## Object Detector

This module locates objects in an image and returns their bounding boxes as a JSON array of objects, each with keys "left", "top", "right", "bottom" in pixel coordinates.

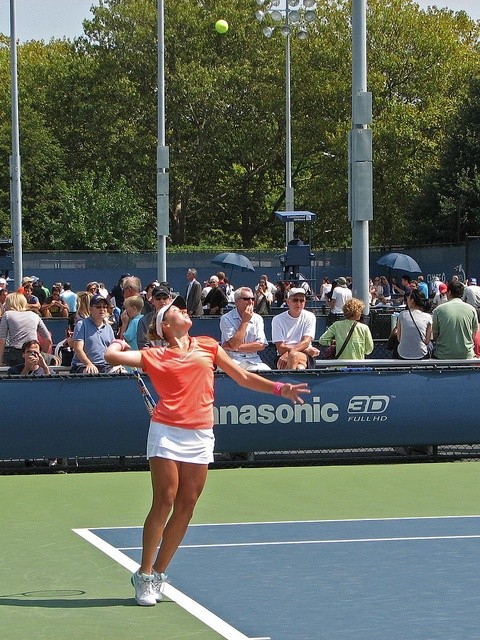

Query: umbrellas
[
  {"left": 211, "top": 252, "right": 256, "bottom": 285},
  {"left": 376, "top": 252, "right": 422, "bottom": 273}
]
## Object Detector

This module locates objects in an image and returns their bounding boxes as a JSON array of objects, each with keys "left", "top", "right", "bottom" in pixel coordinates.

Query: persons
[
  {"left": 320, "top": 277, "right": 352, "bottom": 331},
  {"left": 272, "top": 286, "right": 318, "bottom": 372},
  {"left": 255, "top": 273, "right": 277, "bottom": 314},
  {"left": 214, "top": 272, "right": 230, "bottom": 299},
  {"left": 219, "top": 286, "right": 272, "bottom": 370},
  {"left": 0, "top": 275, "right": 121, "bottom": 310},
  {"left": 69, "top": 294, "right": 125, "bottom": 377},
  {"left": 463, "top": 279, "right": 480, "bottom": 315},
  {"left": 137, "top": 285, "right": 171, "bottom": 348},
  {"left": 394, "top": 289, "right": 432, "bottom": 362},
  {"left": 119, "top": 277, "right": 144, "bottom": 329},
  {"left": 370, "top": 277, "right": 448, "bottom": 303},
  {"left": 319, "top": 297, "right": 375, "bottom": 362},
  {"left": 104, "top": 296, "right": 310, "bottom": 606},
  {"left": 432, "top": 278, "right": 479, "bottom": 365},
  {"left": 1, "top": 295, "right": 52, "bottom": 363},
  {"left": 278, "top": 228, "right": 303, "bottom": 280},
  {"left": 59, "top": 313, "right": 79, "bottom": 366},
  {"left": 142, "top": 279, "right": 161, "bottom": 314},
  {"left": 106, "top": 303, "right": 121, "bottom": 341},
  {"left": 185, "top": 268, "right": 204, "bottom": 317},
  {"left": 118, "top": 296, "right": 145, "bottom": 373},
  {"left": 10, "top": 340, "right": 52, "bottom": 378},
  {"left": 112, "top": 272, "right": 129, "bottom": 308},
  {"left": 275, "top": 280, "right": 313, "bottom": 306},
  {"left": 202, "top": 276, "right": 227, "bottom": 316}
]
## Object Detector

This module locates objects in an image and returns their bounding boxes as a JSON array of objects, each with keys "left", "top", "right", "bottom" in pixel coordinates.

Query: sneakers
[
  {"left": 131, "top": 567, "right": 157, "bottom": 606},
  {"left": 151, "top": 567, "right": 168, "bottom": 601}
]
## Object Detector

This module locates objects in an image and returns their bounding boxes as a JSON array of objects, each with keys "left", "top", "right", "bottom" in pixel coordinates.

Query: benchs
[
  {"left": 0, "top": 365, "right": 72, "bottom": 372},
  {"left": 314, "top": 358, "right": 480, "bottom": 364}
]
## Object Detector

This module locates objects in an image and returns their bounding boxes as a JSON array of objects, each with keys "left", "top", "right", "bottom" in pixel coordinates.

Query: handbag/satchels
[
  {"left": 325, "top": 321, "right": 358, "bottom": 359},
  {"left": 409, "top": 309, "right": 434, "bottom": 359}
]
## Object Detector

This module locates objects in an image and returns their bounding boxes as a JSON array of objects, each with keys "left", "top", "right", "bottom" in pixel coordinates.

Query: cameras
[{"left": 28, "top": 349, "right": 37, "bottom": 362}]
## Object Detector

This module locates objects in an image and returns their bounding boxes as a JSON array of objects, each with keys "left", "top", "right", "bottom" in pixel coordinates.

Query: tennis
[{"left": 215, "top": 20, "right": 228, "bottom": 34}]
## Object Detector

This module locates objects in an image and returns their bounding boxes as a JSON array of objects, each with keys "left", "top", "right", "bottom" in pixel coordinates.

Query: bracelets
[
  {"left": 112, "top": 339, "right": 125, "bottom": 350},
  {"left": 272, "top": 381, "right": 285, "bottom": 396}
]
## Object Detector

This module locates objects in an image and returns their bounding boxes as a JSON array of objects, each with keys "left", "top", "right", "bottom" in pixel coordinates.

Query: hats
[
  {"left": 287, "top": 288, "right": 306, "bottom": 298},
  {"left": 156, "top": 295, "right": 186, "bottom": 339},
  {"left": 334, "top": 277, "right": 347, "bottom": 288},
  {"left": 90, "top": 295, "right": 107, "bottom": 305},
  {"left": 30, "top": 275, "right": 40, "bottom": 284},
  {"left": 22, "top": 277, "right": 32, "bottom": 286},
  {"left": 439, "top": 284, "right": 447, "bottom": 294},
  {"left": 470, "top": 278, "right": 476, "bottom": 284},
  {"left": 152, "top": 286, "right": 170, "bottom": 298}
]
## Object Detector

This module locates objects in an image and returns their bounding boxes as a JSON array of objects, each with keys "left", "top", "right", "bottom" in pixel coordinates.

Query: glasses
[
  {"left": 155, "top": 298, "right": 167, "bottom": 300},
  {"left": 238, "top": 297, "right": 255, "bottom": 301},
  {"left": 91, "top": 305, "right": 108, "bottom": 308},
  {"left": 293, "top": 299, "right": 305, "bottom": 303}
]
